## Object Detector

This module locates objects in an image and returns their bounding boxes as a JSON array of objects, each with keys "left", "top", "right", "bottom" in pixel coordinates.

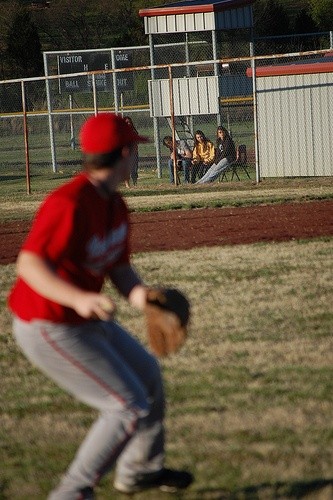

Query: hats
[{"left": 79, "top": 112, "right": 150, "bottom": 156}]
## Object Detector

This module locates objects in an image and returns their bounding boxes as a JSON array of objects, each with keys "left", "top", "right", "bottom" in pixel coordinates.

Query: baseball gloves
[{"left": 146, "top": 286, "right": 190, "bottom": 354}]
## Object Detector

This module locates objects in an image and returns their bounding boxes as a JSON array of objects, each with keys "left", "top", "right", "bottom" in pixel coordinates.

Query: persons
[
  {"left": 6, "top": 114, "right": 195, "bottom": 500},
  {"left": 119, "top": 116, "right": 139, "bottom": 190},
  {"left": 192, "top": 125, "right": 236, "bottom": 185},
  {"left": 188, "top": 130, "right": 215, "bottom": 183},
  {"left": 161, "top": 135, "right": 191, "bottom": 186}
]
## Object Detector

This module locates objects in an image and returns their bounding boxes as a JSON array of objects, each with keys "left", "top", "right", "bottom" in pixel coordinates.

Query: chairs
[{"left": 175, "top": 144, "right": 251, "bottom": 182}]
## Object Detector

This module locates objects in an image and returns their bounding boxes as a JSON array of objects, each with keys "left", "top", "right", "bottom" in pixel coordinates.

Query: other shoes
[{"left": 115, "top": 460, "right": 194, "bottom": 494}]
